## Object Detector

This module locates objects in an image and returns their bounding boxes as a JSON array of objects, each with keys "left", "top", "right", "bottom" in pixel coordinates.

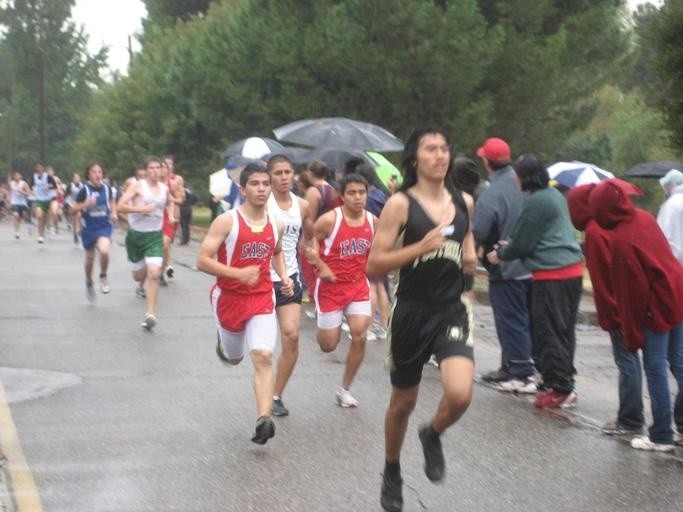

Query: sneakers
[
  {"left": 142, "top": 313, "right": 155, "bottom": 329},
  {"left": 482, "top": 370, "right": 577, "bottom": 408},
  {"left": 99, "top": 274, "right": 110, "bottom": 294},
  {"left": 381, "top": 473, "right": 402, "bottom": 511},
  {"left": 272, "top": 399, "right": 288, "bottom": 416},
  {"left": 603, "top": 421, "right": 674, "bottom": 452},
  {"left": 336, "top": 392, "right": 358, "bottom": 408},
  {"left": 419, "top": 425, "right": 443, "bottom": 481},
  {"left": 252, "top": 416, "right": 273, "bottom": 445}
]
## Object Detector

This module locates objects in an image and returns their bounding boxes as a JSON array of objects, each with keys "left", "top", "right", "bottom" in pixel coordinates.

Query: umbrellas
[{"left": 272, "top": 116, "right": 405, "bottom": 153}]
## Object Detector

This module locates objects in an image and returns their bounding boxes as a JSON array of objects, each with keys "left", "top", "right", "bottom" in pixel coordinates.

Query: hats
[{"left": 476, "top": 138, "right": 510, "bottom": 161}]
